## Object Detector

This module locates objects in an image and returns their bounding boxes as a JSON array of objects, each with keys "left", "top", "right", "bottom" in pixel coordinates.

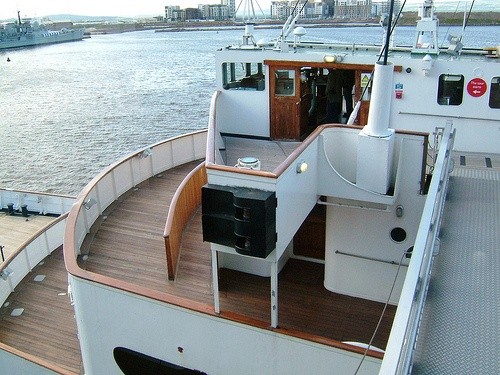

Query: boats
[{"left": 0, "top": 10, "right": 86, "bottom": 49}]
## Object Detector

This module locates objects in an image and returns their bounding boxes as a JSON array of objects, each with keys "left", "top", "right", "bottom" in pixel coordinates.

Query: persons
[{"left": 322, "top": 69, "right": 355, "bottom": 124}]
